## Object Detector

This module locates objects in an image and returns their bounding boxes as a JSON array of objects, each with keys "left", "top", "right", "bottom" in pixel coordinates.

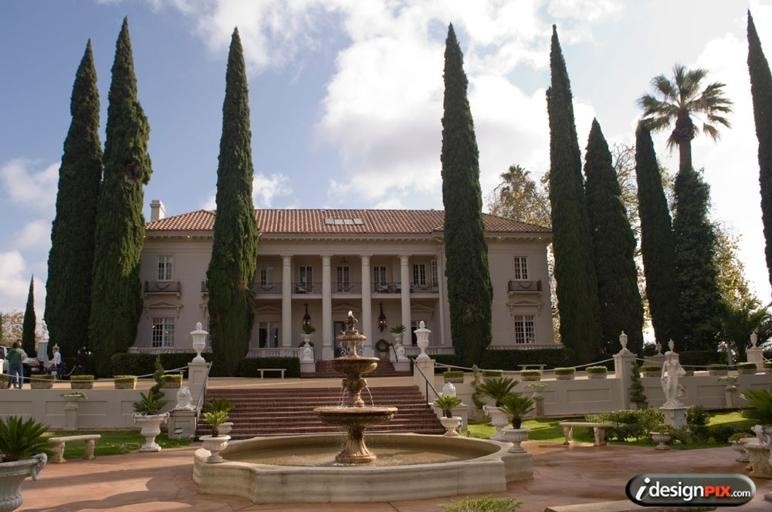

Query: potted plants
[
  {"left": 687, "top": 407, "right": 710, "bottom": 444},
  {"left": 649, "top": 425, "right": 674, "bottom": 450},
  {"left": 728, "top": 387, "right": 772, "bottom": 502},
  {"left": 390, "top": 324, "right": 407, "bottom": 345},
  {"left": 199, "top": 398, "right": 235, "bottom": 464},
  {"left": 433, "top": 394, "right": 462, "bottom": 436},
  {"left": 132, "top": 392, "right": 169, "bottom": 453},
  {"left": 301, "top": 325, "right": 315, "bottom": 346},
  {"left": 479, "top": 377, "right": 535, "bottom": 454},
  {"left": 0, "top": 414, "right": 49, "bottom": 512}
]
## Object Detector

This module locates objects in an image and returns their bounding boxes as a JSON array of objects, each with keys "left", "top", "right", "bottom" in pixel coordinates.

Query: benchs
[
  {"left": 561, "top": 422, "right": 611, "bottom": 448},
  {"left": 50, "top": 434, "right": 101, "bottom": 463},
  {"left": 257, "top": 368, "right": 287, "bottom": 380},
  {"left": 517, "top": 364, "right": 548, "bottom": 371}
]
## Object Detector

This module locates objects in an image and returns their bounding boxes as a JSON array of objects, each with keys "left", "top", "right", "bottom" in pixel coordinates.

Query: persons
[
  {"left": 660, "top": 350, "right": 686, "bottom": 407},
  {"left": 13, "top": 339, "right": 23, "bottom": 388},
  {"left": 42, "top": 344, "right": 63, "bottom": 370},
  {"left": 5, "top": 342, "right": 26, "bottom": 389}
]
